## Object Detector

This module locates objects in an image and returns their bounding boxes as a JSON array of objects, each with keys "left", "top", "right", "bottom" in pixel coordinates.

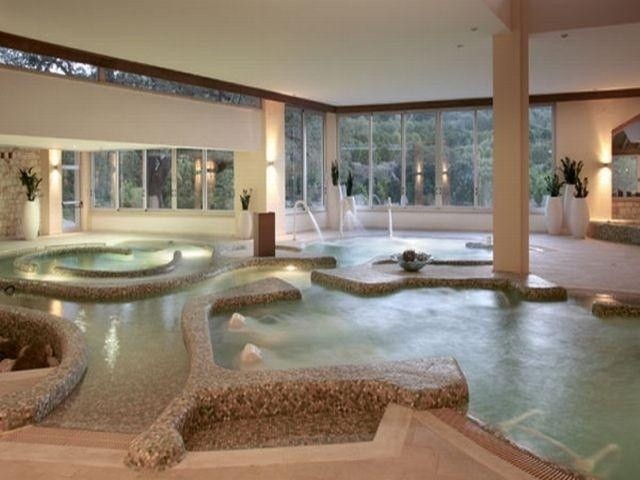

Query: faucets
[
  {"left": 386, "top": 196, "right": 394, "bottom": 231},
  {"left": 292, "top": 199, "right": 309, "bottom": 240}
]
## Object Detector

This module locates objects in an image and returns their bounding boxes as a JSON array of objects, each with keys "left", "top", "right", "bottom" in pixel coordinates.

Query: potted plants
[
  {"left": 326, "top": 159, "right": 356, "bottom": 230},
  {"left": 544, "top": 157, "right": 589, "bottom": 238},
  {"left": 239, "top": 186, "right": 252, "bottom": 240},
  {"left": 17, "top": 164, "right": 43, "bottom": 240}
]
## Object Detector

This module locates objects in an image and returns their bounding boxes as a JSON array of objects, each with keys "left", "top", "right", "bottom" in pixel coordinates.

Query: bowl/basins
[{"left": 391, "top": 254, "right": 433, "bottom": 272}]
